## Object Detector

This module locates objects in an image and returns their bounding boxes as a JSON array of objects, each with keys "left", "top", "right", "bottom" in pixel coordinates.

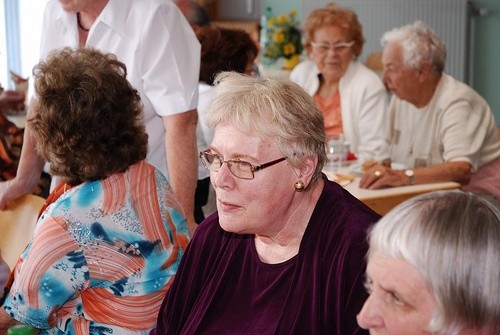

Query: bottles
[{"left": 7, "top": 324, "right": 36, "bottom": 335}]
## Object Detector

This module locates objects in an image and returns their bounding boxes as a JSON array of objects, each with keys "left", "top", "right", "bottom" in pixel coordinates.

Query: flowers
[{"left": 262, "top": 10, "right": 304, "bottom": 59}]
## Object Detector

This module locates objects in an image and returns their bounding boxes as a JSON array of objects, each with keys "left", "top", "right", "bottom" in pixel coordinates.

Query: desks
[{"left": 323, "top": 156, "right": 460, "bottom": 217}]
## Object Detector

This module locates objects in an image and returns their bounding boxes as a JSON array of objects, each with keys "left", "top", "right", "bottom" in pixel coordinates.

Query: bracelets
[{"left": 77, "top": 12, "right": 91, "bottom": 32}]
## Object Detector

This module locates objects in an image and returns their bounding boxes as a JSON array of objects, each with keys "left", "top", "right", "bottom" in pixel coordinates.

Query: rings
[{"left": 375, "top": 171, "right": 381, "bottom": 177}]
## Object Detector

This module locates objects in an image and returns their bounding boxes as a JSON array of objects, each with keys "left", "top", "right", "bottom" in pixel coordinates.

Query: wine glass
[{"left": 326, "top": 131, "right": 343, "bottom": 175}]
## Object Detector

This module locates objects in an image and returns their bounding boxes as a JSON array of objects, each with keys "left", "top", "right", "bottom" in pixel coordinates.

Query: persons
[
  {"left": 0, "top": 0, "right": 221, "bottom": 242},
  {"left": 0, "top": 4, "right": 500, "bottom": 335}
]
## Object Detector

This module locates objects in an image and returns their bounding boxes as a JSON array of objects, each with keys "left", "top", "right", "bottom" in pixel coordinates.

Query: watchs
[{"left": 403, "top": 167, "right": 413, "bottom": 185}]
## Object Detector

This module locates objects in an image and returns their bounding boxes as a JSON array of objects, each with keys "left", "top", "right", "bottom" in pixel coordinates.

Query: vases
[{"left": 283, "top": 55, "right": 300, "bottom": 70}]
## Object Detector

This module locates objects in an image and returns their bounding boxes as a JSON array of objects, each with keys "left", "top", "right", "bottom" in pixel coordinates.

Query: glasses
[
  {"left": 199, "top": 149, "right": 304, "bottom": 180},
  {"left": 246, "top": 62, "right": 259, "bottom": 77},
  {"left": 310, "top": 41, "right": 355, "bottom": 57}
]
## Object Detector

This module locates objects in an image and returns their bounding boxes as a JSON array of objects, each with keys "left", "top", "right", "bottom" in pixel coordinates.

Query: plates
[{"left": 348, "top": 162, "right": 409, "bottom": 176}]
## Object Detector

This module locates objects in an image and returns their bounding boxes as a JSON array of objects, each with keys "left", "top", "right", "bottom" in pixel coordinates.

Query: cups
[
  {"left": 413, "top": 149, "right": 433, "bottom": 171},
  {"left": 343, "top": 128, "right": 355, "bottom": 161}
]
